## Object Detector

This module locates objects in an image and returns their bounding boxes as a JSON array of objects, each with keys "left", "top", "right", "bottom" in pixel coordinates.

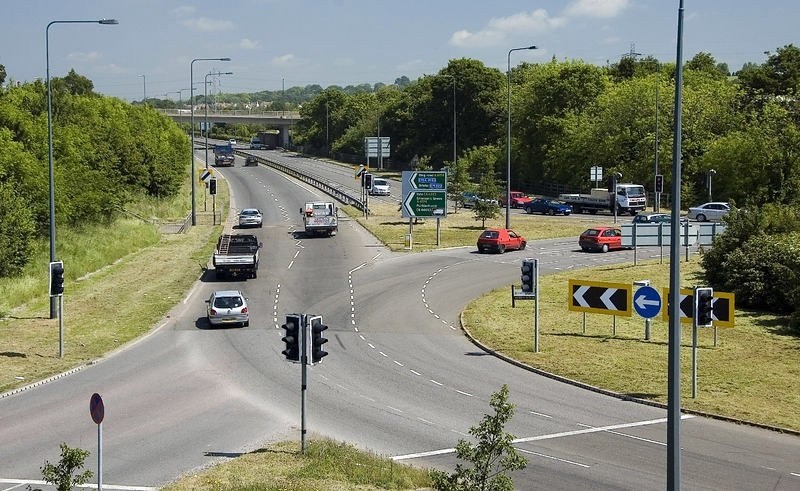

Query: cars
[
  {"left": 454, "top": 182, "right": 749, "bottom": 224},
  {"left": 213, "top": 137, "right": 262, "bottom": 168},
  {"left": 578, "top": 226, "right": 635, "bottom": 254},
  {"left": 477, "top": 228, "right": 527, "bottom": 255},
  {"left": 203, "top": 290, "right": 250, "bottom": 327},
  {"left": 237, "top": 208, "right": 263, "bottom": 228},
  {"left": 368, "top": 177, "right": 391, "bottom": 196}
]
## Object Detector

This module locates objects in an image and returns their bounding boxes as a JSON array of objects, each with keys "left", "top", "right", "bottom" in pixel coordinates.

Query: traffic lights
[
  {"left": 210, "top": 178, "right": 217, "bottom": 195},
  {"left": 281, "top": 315, "right": 302, "bottom": 363},
  {"left": 49, "top": 261, "right": 65, "bottom": 297},
  {"left": 521, "top": 260, "right": 535, "bottom": 293},
  {"left": 654, "top": 175, "right": 664, "bottom": 193},
  {"left": 364, "top": 174, "right": 372, "bottom": 190},
  {"left": 309, "top": 315, "right": 329, "bottom": 365},
  {"left": 695, "top": 287, "right": 713, "bottom": 328}
]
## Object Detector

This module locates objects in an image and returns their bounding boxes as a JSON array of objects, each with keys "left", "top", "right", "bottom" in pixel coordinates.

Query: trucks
[
  {"left": 299, "top": 200, "right": 339, "bottom": 239},
  {"left": 213, "top": 233, "right": 264, "bottom": 280}
]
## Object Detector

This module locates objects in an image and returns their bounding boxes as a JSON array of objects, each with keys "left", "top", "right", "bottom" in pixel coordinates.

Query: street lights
[
  {"left": 204, "top": 72, "right": 235, "bottom": 171},
  {"left": 506, "top": 45, "right": 540, "bottom": 228},
  {"left": 166, "top": 81, "right": 213, "bottom": 144},
  {"left": 137, "top": 74, "right": 146, "bottom": 101},
  {"left": 45, "top": 17, "right": 119, "bottom": 321},
  {"left": 190, "top": 57, "right": 231, "bottom": 228},
  {"left": 436, "top": 74, "right": 458, "bottom": 182}
]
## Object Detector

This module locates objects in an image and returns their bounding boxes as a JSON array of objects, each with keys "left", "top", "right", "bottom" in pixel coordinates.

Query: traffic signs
[{"left": 401, "top": 171, "right": 447, "bottom": 219}]
